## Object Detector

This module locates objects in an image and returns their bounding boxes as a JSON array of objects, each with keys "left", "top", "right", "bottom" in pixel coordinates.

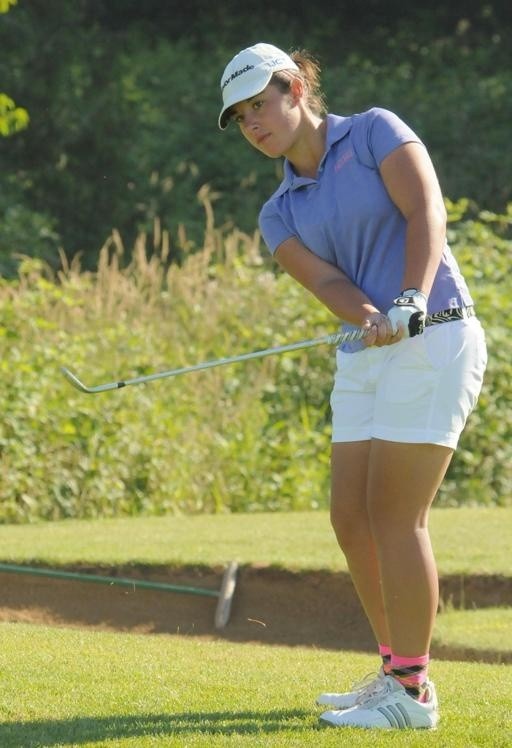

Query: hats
[{"left": 217, "top": 42, "right": 299, "bottom": 131}]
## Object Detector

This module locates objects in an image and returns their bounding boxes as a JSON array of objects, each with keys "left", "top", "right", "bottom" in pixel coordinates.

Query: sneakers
[
  {"left": 318, "top": 675, "right": 437, "bottom": 729},
  {"left": 317, "top": 664, "right": 386, "bottom": 707}
]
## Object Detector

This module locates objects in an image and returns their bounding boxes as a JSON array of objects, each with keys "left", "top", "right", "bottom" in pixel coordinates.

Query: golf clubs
[{"left": 61, "top": 304, "right": 476, "bottom": 393}]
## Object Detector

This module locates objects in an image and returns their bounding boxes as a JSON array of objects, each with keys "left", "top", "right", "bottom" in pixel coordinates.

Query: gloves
[{"left": 387, "top": 288, "right": 428, "bottom": 338}]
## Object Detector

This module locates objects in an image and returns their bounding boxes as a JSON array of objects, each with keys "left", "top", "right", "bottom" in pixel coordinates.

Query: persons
[{"left": 215, "top": 42, "right": 491, "bottom": 731}]
